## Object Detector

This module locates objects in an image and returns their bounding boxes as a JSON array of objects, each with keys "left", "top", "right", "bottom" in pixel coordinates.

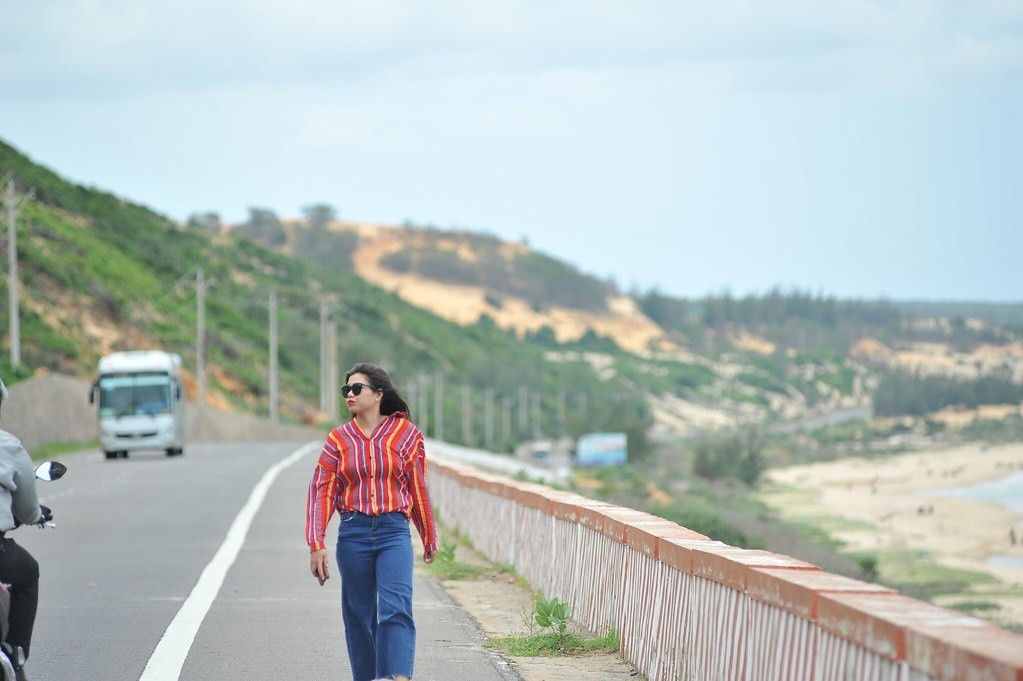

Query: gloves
[{"left": 37, "top": 504, "right": 54, "bottom": 524}]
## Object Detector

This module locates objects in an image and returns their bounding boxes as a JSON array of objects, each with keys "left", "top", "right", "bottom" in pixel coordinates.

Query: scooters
[{"left": 0, "top": 461, "right": 68, "bottom": 643}]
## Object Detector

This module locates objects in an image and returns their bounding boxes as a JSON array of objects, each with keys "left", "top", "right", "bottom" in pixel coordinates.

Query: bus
[{"left": 89, "top": 350, "right": 183, "bottom": 458}]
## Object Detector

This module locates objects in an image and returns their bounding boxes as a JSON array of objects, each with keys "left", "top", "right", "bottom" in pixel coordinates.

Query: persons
[
  {"left": 304, "top": 364, "right": 439, "bottom": 681},
  {"left": 0, "top": 377, "right": 53, "bottom": 669}
]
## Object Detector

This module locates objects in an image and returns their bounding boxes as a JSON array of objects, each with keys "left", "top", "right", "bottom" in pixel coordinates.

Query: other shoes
[{"left": 0, "top": 642, "right": 25, "bottom": 681}]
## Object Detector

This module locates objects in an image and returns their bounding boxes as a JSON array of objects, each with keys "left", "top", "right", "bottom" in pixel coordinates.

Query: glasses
[{"left": 342, "top": 383, "right": 378, "bottom": 398}]
